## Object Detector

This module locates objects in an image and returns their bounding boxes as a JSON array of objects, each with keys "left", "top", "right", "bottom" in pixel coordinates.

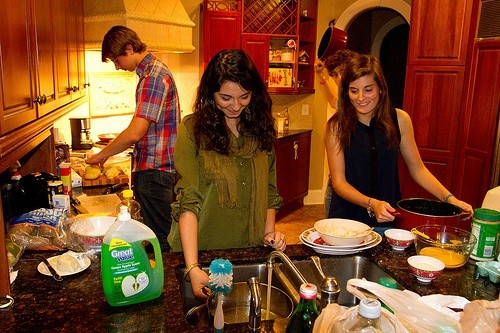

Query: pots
[
  {"left": 317, "top": 16, "right": 348, "bottom": 69},
  {"left": 388, "top": 196, "right": 471, "bottom": 239},
  {"left": 202, "top": 282, "right": 294, "bottom": 325}
]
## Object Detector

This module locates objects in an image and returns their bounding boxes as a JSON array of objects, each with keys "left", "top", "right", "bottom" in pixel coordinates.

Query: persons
[
  {"left": 324, "top": 49, "right": 358, "bottom": 218},
  {"left": 85, "top": 26, "right": 181, "bottom": 252},
  {"left": 326, "top": 55, "right": 473, "bottom": 228},
  {"left": 166, "top": 49, "right": 286, "bottom": 298}
]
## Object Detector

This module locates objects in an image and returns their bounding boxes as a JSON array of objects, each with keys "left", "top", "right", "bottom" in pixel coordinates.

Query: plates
[
  {"left": 37, "top": 255, "right": 91, "bottom": 279},
  {"left": 302, "top": 227, "right": 377, "bottom": 249},
  {"left": 299, "top": 233, "right": 381, "bottom": 255}
]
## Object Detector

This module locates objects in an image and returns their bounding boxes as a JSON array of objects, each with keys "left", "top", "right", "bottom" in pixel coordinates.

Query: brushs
[{"left": 207, "top": 258, "right": 233, "bottom": 333}]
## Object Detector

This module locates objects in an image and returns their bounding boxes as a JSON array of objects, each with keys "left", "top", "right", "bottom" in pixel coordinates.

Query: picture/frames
[{"left": 87, "top": 71, "right": 137, "bottom": 119}]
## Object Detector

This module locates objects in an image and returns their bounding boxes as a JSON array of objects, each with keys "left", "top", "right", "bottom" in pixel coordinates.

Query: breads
[{"left": 6, "top": 210, "right": 78, "bottom": 249}]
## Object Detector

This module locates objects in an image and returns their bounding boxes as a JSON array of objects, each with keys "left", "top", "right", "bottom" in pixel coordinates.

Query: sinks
[
  {"left": 279, "top": 255, "right": 416, "bottom": 311},
  {"left": 173, "top": 262, "right": 301, "bottom": 324}
]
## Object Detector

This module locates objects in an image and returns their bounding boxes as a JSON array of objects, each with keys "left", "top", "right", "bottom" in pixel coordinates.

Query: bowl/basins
[
  {"left": 97, "top": 133, "right": 122, "bottom": 141},
  {"left": 71, "top": 216, "right": 116, "bottom": 245},
  {"left": 313, "top": 217, "right": 477, "bottom": 283}
]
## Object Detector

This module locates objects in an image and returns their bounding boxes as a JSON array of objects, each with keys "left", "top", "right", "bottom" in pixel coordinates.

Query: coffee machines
[{"left": 69, "top": 117, "right": 95, "bottom": 149}]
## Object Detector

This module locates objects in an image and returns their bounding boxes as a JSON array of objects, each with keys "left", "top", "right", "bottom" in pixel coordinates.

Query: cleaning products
[
  {"left": 100, "top": 204, "right": 165, "bottom": 306},
  {"left": 116, "top": 189, "right": 144, "bottom": 223}
]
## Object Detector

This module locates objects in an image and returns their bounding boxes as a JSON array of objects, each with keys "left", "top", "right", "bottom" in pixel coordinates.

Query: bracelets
[
  {"left": 183, "top": 263, "right": 202, "bottom": 283},
  {"left": 366, "top": 197, "right": 375, "bottom": 218},
  {"left": 445, "top": 194, "right": 453, "bottom": 203},
  {"left": 262, "top": 232, "right": 273, "bottom": 240}
]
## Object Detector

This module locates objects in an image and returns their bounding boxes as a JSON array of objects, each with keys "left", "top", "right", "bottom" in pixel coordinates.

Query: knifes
[
  {"left": 70, "top": 194, "right": 89, "bottom": 214},
  {"left": 34, "top": 253, "right": 63, "bottom": 281}
]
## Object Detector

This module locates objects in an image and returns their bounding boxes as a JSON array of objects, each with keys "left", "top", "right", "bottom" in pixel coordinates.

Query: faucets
[
  {"left": 267, "top": 251, "right": 341, "bottom": 315},
  {"left": 245, "top": 276, "right": 262, "bottom": 329}
]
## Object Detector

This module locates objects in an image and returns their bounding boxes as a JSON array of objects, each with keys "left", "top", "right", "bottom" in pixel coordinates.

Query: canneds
[{"left": 465, "top": 208, "right": 500, "bottom": 262}]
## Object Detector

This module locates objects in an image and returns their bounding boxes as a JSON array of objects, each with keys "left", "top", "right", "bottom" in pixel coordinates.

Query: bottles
[
  {"left": 101, "top": 206, "right": 164, "bottom": 307},
  {"left": 346, "top": 297, "right": 382, "bottom": 333},
  {"left": 284, "top": 107, "right": 289, "bottom": 130},
  {"left": 285, "top": 283, "right": 320, "bottom": 333},
  {"left": 468, "top": 208, "right": 500, "bottom": 259},
  {"left": 115, "top": 189, "right": 145, "bottom": 247},
  {"left": 375, "top": 277, "right": 397, "bottom": 315}
]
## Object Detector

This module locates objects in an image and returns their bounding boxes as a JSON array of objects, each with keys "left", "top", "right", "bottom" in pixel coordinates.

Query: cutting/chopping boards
[
  {"left": 76, "top": 194, "right": 121, "bottom": 217},
  {"left": 78, "top": 166, "right": 130, "bottom": 189}
]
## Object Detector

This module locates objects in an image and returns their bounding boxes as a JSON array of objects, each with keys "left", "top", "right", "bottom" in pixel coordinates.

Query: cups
[{"left": 281, "top": 47, "right": 294, "bottom": 62}]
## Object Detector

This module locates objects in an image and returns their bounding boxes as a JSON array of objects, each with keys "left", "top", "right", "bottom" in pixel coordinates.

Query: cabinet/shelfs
[
  {"left": 275, "top": 133, "right": 309, "bottom": 223},
  {"left": 0, "top": 0, "right": 87, "bottom": 174},
  {"left": 397, "top": 0, "right": 500, "bottom": 208},
  {"left": 199, "top": 0, "right": 318, "bottom": 96}
]
